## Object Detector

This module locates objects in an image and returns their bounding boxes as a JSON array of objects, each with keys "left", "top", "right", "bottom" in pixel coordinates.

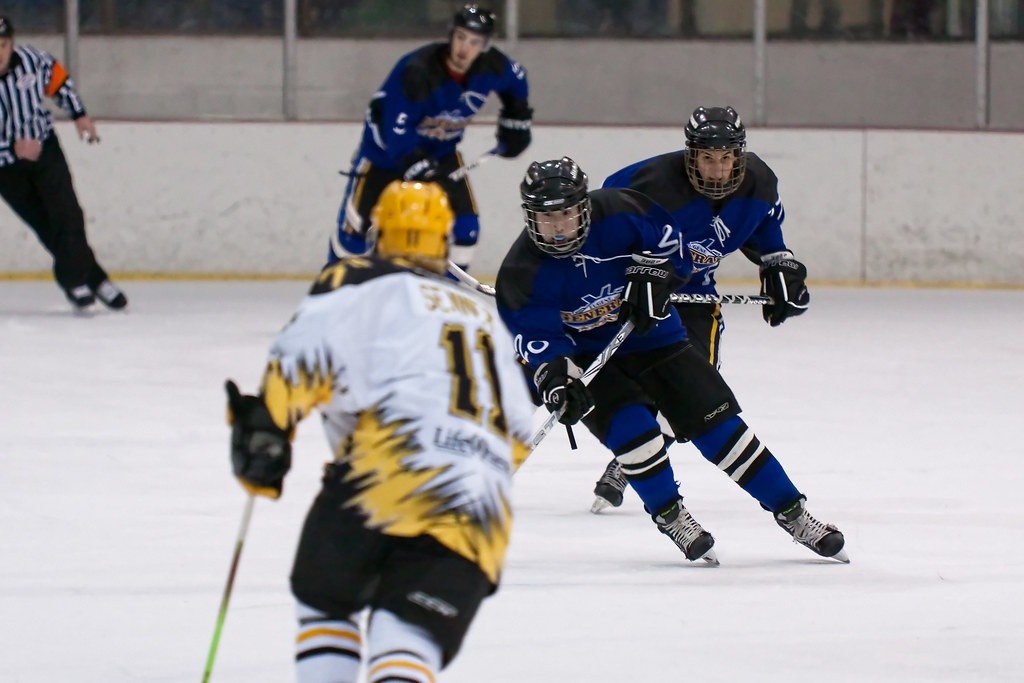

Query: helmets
[
  {"left": 520, "top": 157, "right": 592, "bottom": 260},
  {"left": 684, "top": 106, "right": 746, "bottom": 200},
  {"left": 448, "top": 5, "right": 496, "bottom": 52},
  {"left": 369, "top": 179, "right": 453, "bottom": 259},
  {"left": 0, "top": 15, "right": 13, "bottom": 36}
]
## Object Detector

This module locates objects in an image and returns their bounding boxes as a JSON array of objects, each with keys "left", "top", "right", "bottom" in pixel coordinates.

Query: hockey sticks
[
  {"left": 201, "top": 487, "right": 261, "bottom": 683},
  {"left": 441, "top": 149, "right": 504, "bottom": 194},
  {"left": 668, "top": 290, "right": 780, "bottom": 305},
  {"left": 514, "top": 317, "right": 636, "bottom": 461},
  {"left": 448, "top": 256, "right": 494, "bottom": 295}
]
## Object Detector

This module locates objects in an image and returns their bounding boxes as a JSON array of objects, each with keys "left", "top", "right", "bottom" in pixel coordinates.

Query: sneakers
[
  {"left": 592, "top": 457, "right": 628, "bottom": 513},
  {"left": 760, "top": 489, "right": 851, "bottom": 563},
  {"left": 644, "top": 493, "right": 719, "bottom": 565}
]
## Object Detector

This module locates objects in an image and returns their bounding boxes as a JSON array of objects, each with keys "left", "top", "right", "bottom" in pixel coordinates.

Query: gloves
[
  {"left": 401, "top": 152, "right": 444, "bottom": 184},
  {"left": 498, "top": 109, "right": 532, "bottom": 156},
  {"left": 758, "top": 259, "right": 809, "bottom": 326},
  {"left": 532, "top": 357, "right": 596, "bottom": 424},
  {"left": 619, "top": 254, "right": 677, "bottom": 327}
]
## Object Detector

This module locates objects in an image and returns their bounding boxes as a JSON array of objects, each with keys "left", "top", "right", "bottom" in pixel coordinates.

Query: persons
[
  {"left": 0, "top": 15, "right": 127, "bottom": 314},
  {"left": 222, "top": 180, "right": 533, "bottom": 683},
  {"left": 327, "top": 3, "right": 534, "bottom": 283},
  {"left": 494, "top": 156, "right": 850, "bottom": 566},
  {"left": 590, "top": 105, "right": 810, "bottom": 515}
]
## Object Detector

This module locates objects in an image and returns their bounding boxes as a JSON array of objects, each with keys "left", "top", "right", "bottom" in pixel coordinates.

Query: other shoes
[
  {"left": 94, "top": 279, "right": 127, "bottom": 307},
  {"left": 66, "top": 282, "right": 94, "bottom": 308}
]
